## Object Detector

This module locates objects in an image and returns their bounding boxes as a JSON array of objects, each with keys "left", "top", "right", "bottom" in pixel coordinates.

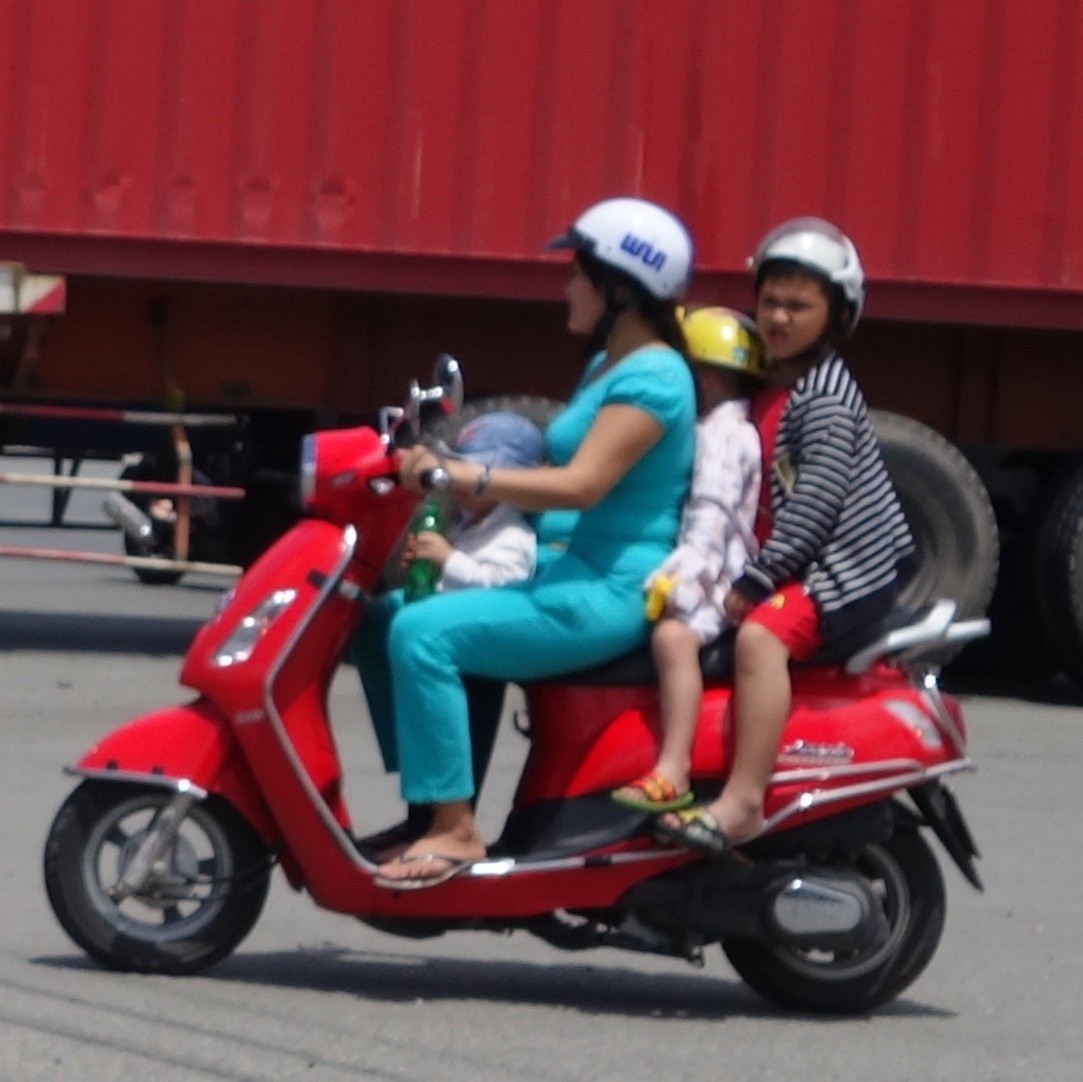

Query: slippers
[
  {"left": 361, "top": 821, "right": 413, "bottom": 850},
  {"left": 613, "top": 777, "right": 698, "bottom": 812},
  {"left": 655, "top": 804, "right": 755, "bottom": 870},
  {"left": 372, "top": 842, "right": 474, "bottom": 889}
]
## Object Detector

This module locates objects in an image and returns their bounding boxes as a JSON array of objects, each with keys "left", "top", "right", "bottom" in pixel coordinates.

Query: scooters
[
  {"left": 107, "top": 409, "right": 379, "bottom": 586},
  {"left": 43, "top": 348, "right": 994, "bottom": 1021}
]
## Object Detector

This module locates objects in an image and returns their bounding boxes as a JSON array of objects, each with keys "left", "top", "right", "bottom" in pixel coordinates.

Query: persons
[
  {"left": 610, "top": 303, "right": 766, "bottom": 812},
  {"left": 346, "top": 197, "right": 695, "bottom": 887},
  {"left": 374, "top": 414, "right": 545, "bottom": 846},
  {"left": 655, "top": 219, "right": 914, "bottom": 875}
]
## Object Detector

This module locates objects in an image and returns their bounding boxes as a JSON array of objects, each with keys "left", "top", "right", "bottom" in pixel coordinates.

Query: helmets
[
  {"left": 752, "top": 217, "right": 863, "bottom": 334},
  {"left": 436, "top": 410, "right": 542, "bottom": 478},
  {"left": 550, "top": 196, "right": 693, "bottom": 304},
  {"left": 683, "top": 307, "right": 766, "bottom": 381}
]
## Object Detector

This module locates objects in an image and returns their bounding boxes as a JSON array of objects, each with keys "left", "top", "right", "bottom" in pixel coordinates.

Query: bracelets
[{"left": 473, "top": 466, "right": 490, "bottom": 496}]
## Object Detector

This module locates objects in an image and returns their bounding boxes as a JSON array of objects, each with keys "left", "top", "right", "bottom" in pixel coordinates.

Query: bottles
[
  {"left": 646, "top": 578, "right": 665, "bottom": 620},
  {"left": 405, "top": 499, "right": 443, "bottom": 605}
]
